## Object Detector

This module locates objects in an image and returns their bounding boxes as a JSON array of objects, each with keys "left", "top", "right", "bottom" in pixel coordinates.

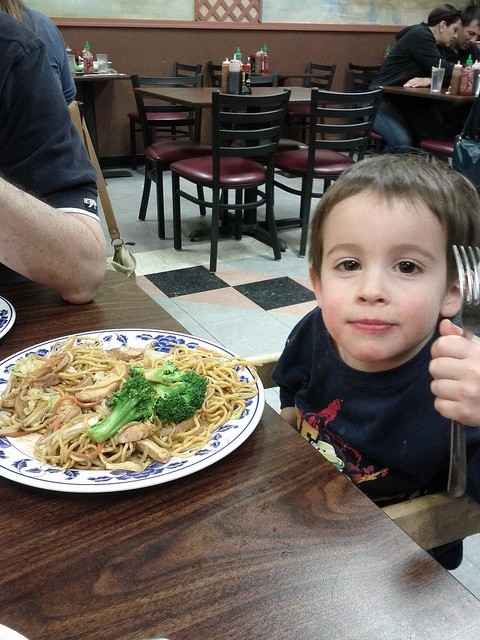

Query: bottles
[
  {"left": 462, "top": 67, "right": 474, "bottom": 95},
  {"left": 234, "top": 46, "right": 242, "bottom": 60},
  {"left": 75, "top": 50, "right": 84, "bottom": 76},
  {"left": 245, "top": 44, "right": 269, "bottom": 77},
  {"left": 450, "top": 64, "right": 461, "bottom": 96},
  {"left": 66, "top": 43, "right": 76, "bottom": 77},
  {"left": 228, "top": 55, "right": 241, "bottom": 95},
  {"left": 82, "top": 40, "right": 95, "bottom": 75},
  {"left": 241, "top": 64, "right": 252, "bottom": 95},
  {"left": 221, "top": 57, "right": 229, "bottom": 93}
]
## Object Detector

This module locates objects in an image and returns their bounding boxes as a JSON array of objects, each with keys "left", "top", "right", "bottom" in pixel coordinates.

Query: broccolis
[
  {"left": 129, "top": 360, "right": 208, "bottom": 425},
  {"left": 88, "top": 376, "right": 156, "bottom": 442}
]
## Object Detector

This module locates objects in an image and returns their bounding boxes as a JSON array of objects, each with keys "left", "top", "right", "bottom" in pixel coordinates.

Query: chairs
[
  {"left": 126, "top": 60, "right": 203, "bottom": 171},
  {"left": 207, "top": 59, "right": 247, "bottom": 148},
  {"left": 274, "top": 83, "right": 386, "bottom": 259},
  {"left": 346, "top": 61, "right": 386, "bottom": 154},
  {"left": 169, "top": 87, "right": 293, "bottom": 275},
  {"left": 417, "top": 88, "right": 480, "bottom": 169},
  {"left": 127, "top": 71, "right": 207, "bottom": 241},
  {"left": 287, "top": 60, "right": 338, "bottom": 149}
]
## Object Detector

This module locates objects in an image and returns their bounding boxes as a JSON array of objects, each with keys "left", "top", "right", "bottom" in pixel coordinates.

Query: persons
[
  {"left": 442, "top": 5, "right": 480, "bottom": 65},
  {"left": 272, "top": 157, "right": 480, "bottom": 570},
  {"left": 362, "top": 4, "right": 462, "bottom": 155},
  {"left": 0, "top": 0, "right": 77, "bottom": 105},
  {"left": 0, "top": 14, "right": 106, "bottom": 305}
]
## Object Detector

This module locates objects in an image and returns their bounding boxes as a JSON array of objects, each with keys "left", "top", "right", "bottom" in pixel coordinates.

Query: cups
[
  {"left": 97, "top": 54, "right": 110, "bottom": 74},
  {"left": 431, "top": 67, "right": 445, "bottom": 95}
]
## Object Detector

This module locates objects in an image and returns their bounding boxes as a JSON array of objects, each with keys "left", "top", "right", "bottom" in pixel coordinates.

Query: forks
[{"left": 446, "top": 244, "right": 480, "bottom": 499}]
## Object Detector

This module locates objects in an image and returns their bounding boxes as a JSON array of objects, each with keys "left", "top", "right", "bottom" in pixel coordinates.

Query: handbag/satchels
[
  {"left": 67, "top": 101, "right": 138, "bottom": 284},
  {"left": 451, "top": 92, "right": 480, "bottom": 191}
]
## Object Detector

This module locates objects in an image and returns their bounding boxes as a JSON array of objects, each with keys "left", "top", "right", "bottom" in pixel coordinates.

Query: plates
[{"left": 0, "top": 293, "right": 16, "bottom": 339}]
